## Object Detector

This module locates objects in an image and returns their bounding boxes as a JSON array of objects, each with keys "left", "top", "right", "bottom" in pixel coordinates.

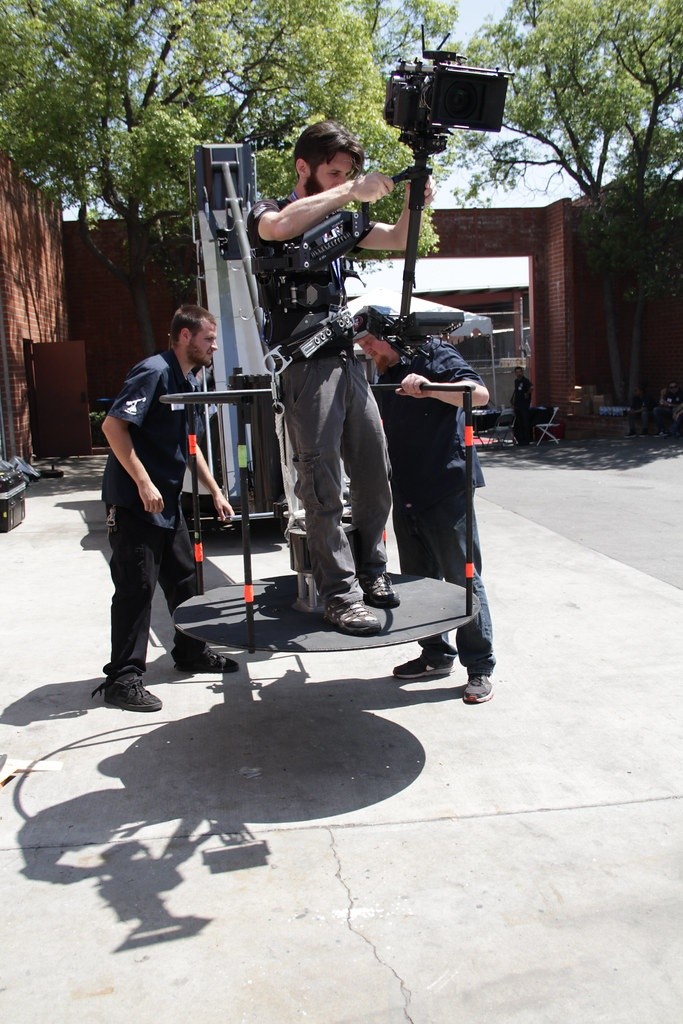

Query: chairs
[
  {"left": 473, "top": 411, "right": 518, "bottom": 449},
  {"left": 534, "top": 406, "right": 561, "bottom": 447}
]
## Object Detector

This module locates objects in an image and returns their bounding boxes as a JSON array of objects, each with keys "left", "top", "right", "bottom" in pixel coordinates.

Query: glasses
[{"left": 669, "top": 385, "right": 677, "bottom": 389}]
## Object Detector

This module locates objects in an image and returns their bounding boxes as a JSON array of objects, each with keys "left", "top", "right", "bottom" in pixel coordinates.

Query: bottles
[{"left": 599, "top": 406, "right": 630, "bottom": 416}]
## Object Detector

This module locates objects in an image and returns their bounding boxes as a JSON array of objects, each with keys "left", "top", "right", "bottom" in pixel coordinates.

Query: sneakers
[
  {"left": 360, "top": 573, "right": 401, "bottom": 608},
  {"left": 393, "top": 658, "right": 454, "bottom": 679},
  {"left": 174, "top": 652, "right": 238, "bottom": 673},
  {"left": 324, "top": 601, "right": 382, "bottom": 636},
  {"left": 91, "top": 672, "right": 163, "bottom": 711},
  {"left": 464, "top": 674, "right": 494, "bottom": 702}
]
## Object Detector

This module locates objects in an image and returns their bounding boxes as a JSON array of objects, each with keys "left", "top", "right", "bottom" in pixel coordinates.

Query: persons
[
  {"left": 351, "top": 305, "right": 496, "bottom": 703},
  {"left": 247, "top": 120, "right": 437, "bottom": 635},
  {"left": 624, "top": 381, "right": 683, "bottom": 438},
  {"left": 510, "top": 366, "right": 534, "bottom": 446},
  {"left": 102, "top": 305, "right": 236, "bottom": 712}
]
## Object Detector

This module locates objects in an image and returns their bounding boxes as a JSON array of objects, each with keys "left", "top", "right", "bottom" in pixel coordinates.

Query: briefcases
[{"left": 0, "top": 468, "right": 26, "bottom": 533}]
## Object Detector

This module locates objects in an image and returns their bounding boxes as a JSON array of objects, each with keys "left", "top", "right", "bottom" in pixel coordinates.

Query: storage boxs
[
  {"left": 570, "top": 386, "right": 614, "bottom": 415},
  {"left": 0, "top": 469, "right": 26, "bottom": 532}
]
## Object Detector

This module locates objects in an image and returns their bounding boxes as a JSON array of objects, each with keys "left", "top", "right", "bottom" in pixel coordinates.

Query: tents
[{"left": 347, "top": 290, "right": 500, "bottom": 443}]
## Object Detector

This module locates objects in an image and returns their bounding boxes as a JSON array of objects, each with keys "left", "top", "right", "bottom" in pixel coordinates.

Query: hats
[{"left": 349, "top": 304, "right": 391, "bottom": 341}]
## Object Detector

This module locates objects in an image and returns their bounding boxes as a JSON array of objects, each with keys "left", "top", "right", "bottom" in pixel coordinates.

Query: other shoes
[
  {"left": 624, "top": 430, "right": 637, "bottom": 438},
  {"left": 652, "top": 429, "right": 665, "bottom": 437},
  {"left": 639, "top": 429, "right": 649, "bottom": 437}
]
